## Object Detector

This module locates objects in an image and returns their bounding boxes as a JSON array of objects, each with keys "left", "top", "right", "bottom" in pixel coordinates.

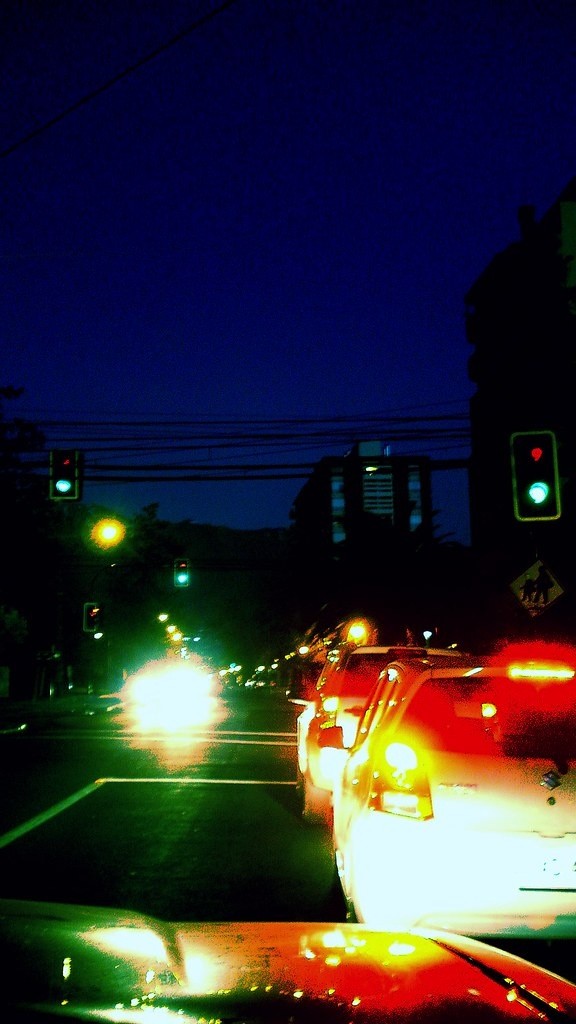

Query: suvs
[
  {"left": 295, "top": 646, "right": 467, "bottom": 822},
  {"left": 334, "top": 656, "right": 576, "bottom": 942}
]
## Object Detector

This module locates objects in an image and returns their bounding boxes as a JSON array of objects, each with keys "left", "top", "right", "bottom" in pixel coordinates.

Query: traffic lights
[
  {"left": 83, "top": 602, "right": 99, "bottom": 632},
  {"left": 509, "top": 430, "right": 561, "bottom": 520},
  {"left": 48, "top": 448, "right": 80, "bottom": 500},
  {"left": 174, "top": 557, "right": 190, "bottom": 587}
]
